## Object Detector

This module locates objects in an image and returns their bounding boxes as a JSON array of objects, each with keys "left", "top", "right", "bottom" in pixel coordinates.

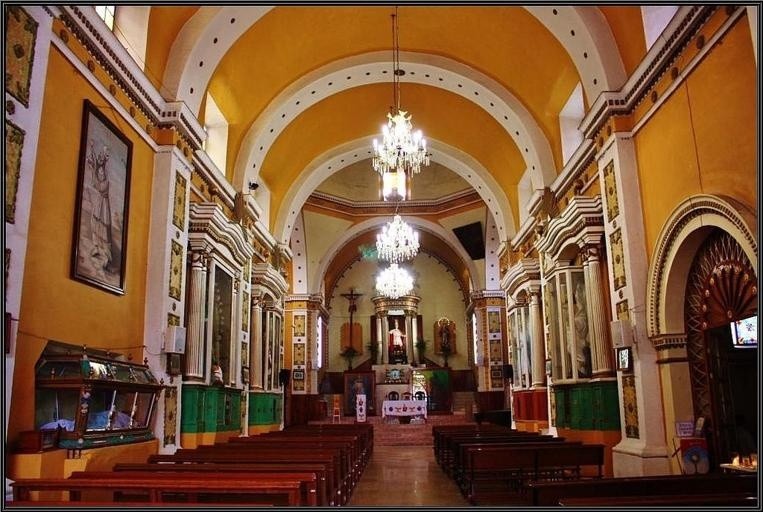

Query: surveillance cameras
[{"left": 249, "top": 176, "right": 259, "bottom": 190}]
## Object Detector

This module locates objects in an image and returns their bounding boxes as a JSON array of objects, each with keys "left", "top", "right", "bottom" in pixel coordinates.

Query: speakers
[
  {"left": 279, "top": 368, "right": 291, "bottom": 381},
  {"left": 609, "top": 319, "right": 633, "bottom": 349},
  {"left": 162, "top": 324, "right": 188, "bottom": 355},
  {"left": 502, "top": 364, "right": 514, "bottom": 380}
]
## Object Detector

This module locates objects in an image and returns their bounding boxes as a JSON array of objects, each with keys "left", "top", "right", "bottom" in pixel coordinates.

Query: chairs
[{"left": 388, "top": 390, "right": 425, "bottom": 401}]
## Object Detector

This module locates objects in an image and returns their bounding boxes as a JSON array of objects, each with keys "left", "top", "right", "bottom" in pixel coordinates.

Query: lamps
[{"left": 371, "top": 4, "right": 432, "bottom": 301}]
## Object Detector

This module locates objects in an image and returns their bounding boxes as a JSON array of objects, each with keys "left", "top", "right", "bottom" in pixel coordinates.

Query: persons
[
  {"left": 388, "top": 320, "right": 406, "bottom": 359},
  {"left": 90, "top": 155, "right": 112, "bottom": 276},
  {"left": 344, "top": 295, "right": 359, "bottom": 313}
]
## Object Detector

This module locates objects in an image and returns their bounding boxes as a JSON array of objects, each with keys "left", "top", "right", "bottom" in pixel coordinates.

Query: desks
[{"left": 381, "top": 399, "right": 428, "bottom": 424}]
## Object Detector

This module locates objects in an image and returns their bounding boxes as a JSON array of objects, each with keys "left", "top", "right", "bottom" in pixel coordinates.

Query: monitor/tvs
[{"left": 728, "top": 315, "right": 758, "bottom": 351}]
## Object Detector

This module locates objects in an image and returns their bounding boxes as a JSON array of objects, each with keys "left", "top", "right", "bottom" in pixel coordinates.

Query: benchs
[
  {"left": 431, "top": 421, "right": 759, "bottom": 508},
  {"left": 2, "top": 421, "right": 372, "bottom": 512}
]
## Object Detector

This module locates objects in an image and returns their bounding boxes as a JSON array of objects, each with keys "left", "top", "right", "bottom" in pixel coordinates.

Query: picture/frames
[{"left": 68, "top": 99, "right": 132, "bottom": 298}]
